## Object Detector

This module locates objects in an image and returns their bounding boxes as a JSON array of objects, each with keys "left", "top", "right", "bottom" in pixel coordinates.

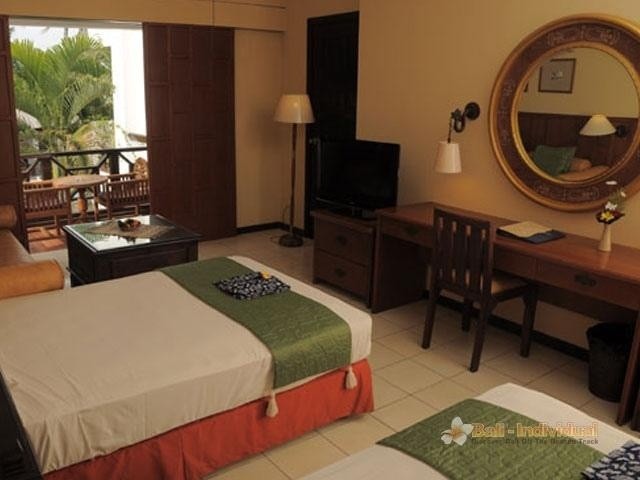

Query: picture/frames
[{"left": 538, "top": 58, "right": 576, "bottom": 94}]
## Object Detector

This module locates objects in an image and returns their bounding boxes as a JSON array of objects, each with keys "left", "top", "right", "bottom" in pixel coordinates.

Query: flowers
[{"left": 596, "top": 180, "right": 628, "bottom": 225}]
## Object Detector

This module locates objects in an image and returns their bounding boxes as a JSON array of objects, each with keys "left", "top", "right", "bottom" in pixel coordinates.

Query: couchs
[{"left": 0, "top": 204, "right": 65, "bottom": 300}]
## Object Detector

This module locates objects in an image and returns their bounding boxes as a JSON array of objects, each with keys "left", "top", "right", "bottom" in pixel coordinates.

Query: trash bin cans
[{"left": 586, "top": 321, "right": 639, "bottom": 403}]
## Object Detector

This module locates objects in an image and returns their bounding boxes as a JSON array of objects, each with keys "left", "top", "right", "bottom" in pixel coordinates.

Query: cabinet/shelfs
[{"left": 310, "top": 208, "right": 376, "bottom": 308}]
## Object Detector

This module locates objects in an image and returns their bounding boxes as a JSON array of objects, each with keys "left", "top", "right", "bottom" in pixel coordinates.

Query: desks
[
  {"left": 52, "top": 174, "right": 108, "bottom": 224},
  {"left": 370, "top": 203, "right": 640, "bottom": 425}
]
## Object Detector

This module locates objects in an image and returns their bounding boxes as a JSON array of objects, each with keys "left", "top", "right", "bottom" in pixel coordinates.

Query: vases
[{"left": 598, "top": 224, "right": 611, "bottom": 251}]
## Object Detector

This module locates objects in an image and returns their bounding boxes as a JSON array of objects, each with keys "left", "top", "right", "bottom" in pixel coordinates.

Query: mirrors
[{"left": 487, "top": 12, "right": 640, "bottom": 213}]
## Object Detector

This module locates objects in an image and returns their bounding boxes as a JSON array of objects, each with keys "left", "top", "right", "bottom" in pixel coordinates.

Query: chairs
[
  {"left": 22, "top": 181, "right": 73, "bottom": 236},
  {"left": 422, "top": 206, "right": 541, "bottom": 372},
  {"left": 94, "top": 170, "right": 151, "bottom": 221}
]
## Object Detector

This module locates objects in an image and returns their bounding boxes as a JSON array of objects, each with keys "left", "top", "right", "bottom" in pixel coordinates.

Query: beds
[
  {"left": 0, "top": 255, "right": 373, "bottom": 480},
  {"left": 304, "top": 383, "right": 640, "bottom": 480}
]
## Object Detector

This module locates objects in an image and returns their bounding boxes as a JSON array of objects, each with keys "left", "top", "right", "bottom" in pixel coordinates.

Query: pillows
[{"left": 532, "top": 145, "right": 576, "bottom": 177}]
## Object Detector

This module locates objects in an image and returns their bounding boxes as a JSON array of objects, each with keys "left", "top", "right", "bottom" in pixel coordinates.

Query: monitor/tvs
[{"left": 313, "top": 136, "right": 400, "bottom": 222}]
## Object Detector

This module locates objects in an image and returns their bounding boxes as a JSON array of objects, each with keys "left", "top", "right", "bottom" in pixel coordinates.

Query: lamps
[
  {"left": 580, "top": 114, "right": 616, "bottom": 166},
  {"left": 434, "top": 102, "right": 480, "bottom": 174},
  {"left": 273, "top": 94, "right": 316, "bottom": 247}
]
